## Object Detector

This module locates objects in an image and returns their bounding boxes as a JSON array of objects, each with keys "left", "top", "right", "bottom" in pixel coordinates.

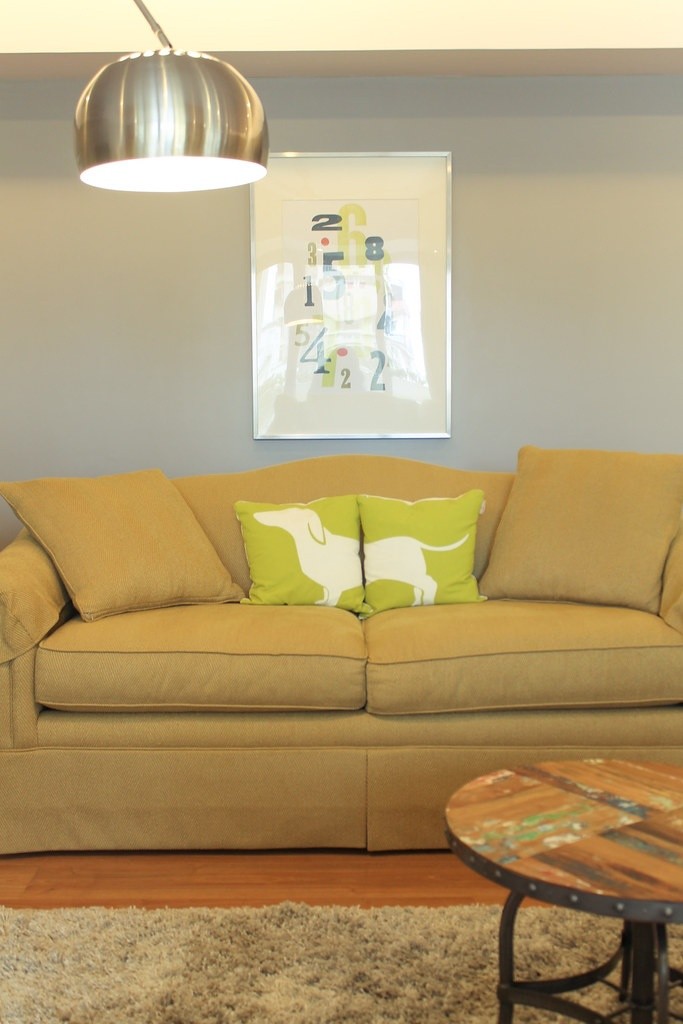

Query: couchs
[{"left": 0, "top": 455, "right": 682, "bottom": 853}]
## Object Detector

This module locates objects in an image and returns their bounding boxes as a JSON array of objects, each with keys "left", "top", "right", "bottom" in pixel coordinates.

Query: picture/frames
[{"left": 249, "top": 151, "right": 453, "bottom": 440}]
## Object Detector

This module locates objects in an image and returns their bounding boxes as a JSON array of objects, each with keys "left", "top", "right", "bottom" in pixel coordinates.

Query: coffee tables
[{"left": 442, "top": 758, "right": 683, "bottom": 1024}]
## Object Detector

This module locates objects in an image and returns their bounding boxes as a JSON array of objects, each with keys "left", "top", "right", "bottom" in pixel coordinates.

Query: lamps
[{"left": 73, "top": 0, "right": 270, "bottom": 193}]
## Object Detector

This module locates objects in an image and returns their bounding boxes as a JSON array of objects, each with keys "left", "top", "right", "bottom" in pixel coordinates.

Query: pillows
[
  {"left": 479, "top": 445, "right": 683, "bottom": 616},
  {"left": 0, "top": 467, "right": 245, "bottom": 622},
  {"left": 232, "top": 494, "right": 375, "bottom": 614},
  {"left": 356, "top": 489, "right": 489, "bottom": 619}
]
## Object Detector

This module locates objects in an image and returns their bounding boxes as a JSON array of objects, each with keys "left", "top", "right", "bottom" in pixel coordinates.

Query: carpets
[{"left": 0, "top": 903, "right": 683, "bottom": 1024}]
